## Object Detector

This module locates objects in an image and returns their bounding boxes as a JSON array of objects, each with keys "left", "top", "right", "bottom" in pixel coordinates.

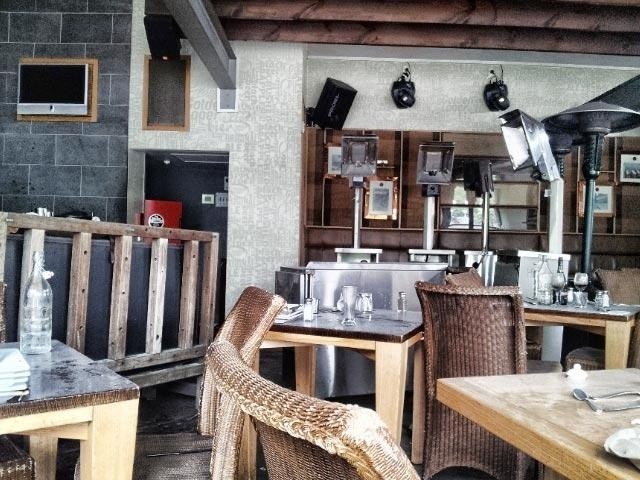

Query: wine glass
[{"left": 574, "top": 272, "right": 588, "bottom": 308}]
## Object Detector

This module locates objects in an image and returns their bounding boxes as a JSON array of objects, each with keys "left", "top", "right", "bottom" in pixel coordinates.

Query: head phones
[
  {"left": 396, "top": 80, "right": 415, "bottom": 108},
  {"left": 491, "top": 82, "right": 510, "bottom": 111}
]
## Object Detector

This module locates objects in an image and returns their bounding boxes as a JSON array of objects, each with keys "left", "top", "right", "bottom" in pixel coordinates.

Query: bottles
[
  {"left": 566, "top": 287, "right": 575, "bottom": 303},
  {"left": 304, "top": 298, "right": 313, "bottom": 321},
  {"left": 19, "top": 250, "right": 53, "bottom": 355},
  {"left": 534, "top": 254, "right": 566, "bottom": 305},
  {"left": 398, "top": 292, "right": 407, "bottom": 312},
  {"left": 596, "top": 291, "right": 610, "bottom": 311},
  {"left": 566, "top": 364, "right": 587, "bottom": 391}
]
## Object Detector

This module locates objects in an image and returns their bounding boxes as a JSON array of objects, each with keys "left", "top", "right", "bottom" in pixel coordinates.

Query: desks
[
  {"left": 524, "top": 296, "right": 639, "bottom": 368},
  {"left": 434, "top": 368, "right": 640, "bottom": 480}
]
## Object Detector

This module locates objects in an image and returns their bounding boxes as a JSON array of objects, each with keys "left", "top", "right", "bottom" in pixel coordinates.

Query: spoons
[{"left": 573, "top": 388, "right": 602, "bottom": 413}]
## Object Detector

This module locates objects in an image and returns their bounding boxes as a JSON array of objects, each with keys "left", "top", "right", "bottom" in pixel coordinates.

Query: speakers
[
  {"left": 312, "top": 78, "right": 357, "bottom": 130},
  {"left": 144, "top": 14, "right": 181, "bottom": 62}
]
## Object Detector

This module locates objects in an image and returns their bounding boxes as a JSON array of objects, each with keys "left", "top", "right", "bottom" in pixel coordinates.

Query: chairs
[
  {"left": 564, "top": 344, "right": 637, "bottom": 373},
  {"left": 591, "top": 268, "right": 639, "bottom": 305},
  {"left": 204, "top": 337, "right": 421, "bottom": 480},
  {"left": 74, "top": 285, "right": 286, "bottom": 480},
  {"left": 415, "top": 280, "right": 564, "bottom": 479},
  {"left": 444, "top": 268, "right": 543, "bottom": 361}
]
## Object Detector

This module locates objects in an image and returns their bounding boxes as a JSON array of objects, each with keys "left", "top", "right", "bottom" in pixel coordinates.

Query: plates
[{"left": 0, "top": 348, "right": 31, "bottom": 404}]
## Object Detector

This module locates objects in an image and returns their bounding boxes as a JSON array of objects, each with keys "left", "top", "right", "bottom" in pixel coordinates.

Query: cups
[
  {"left": 342, "top": 286, "right": 358, "bottom": 326},
  {"left": 358, "top": 293, "right": 374, "bottom": 318}
]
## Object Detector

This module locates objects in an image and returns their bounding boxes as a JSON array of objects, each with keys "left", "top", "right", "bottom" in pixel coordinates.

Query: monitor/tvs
[{"left": 17, "top": 63, "right": 88, "bottom": 116}]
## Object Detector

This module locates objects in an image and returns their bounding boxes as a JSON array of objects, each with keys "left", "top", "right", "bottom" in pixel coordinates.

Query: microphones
[
  {"left": 490, "top": 70, "right": 498, "bottom": 84},
  {"left": 405, "top": 68, "right": 412, "bottom": 88}
]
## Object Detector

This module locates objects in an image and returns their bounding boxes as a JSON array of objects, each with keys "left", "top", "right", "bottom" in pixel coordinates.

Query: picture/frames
[
  {"left": 617, "top": 150, "right": 640, "bottom": 186},
  {"left": 365, "top": 175, "right": 399, "bottom": 222},
  {"left": 322, "top": 142, "right": 347, "bottom": 180},
  {"left": 578, "top": 178, "right": 617, "bottom": 218}
]
